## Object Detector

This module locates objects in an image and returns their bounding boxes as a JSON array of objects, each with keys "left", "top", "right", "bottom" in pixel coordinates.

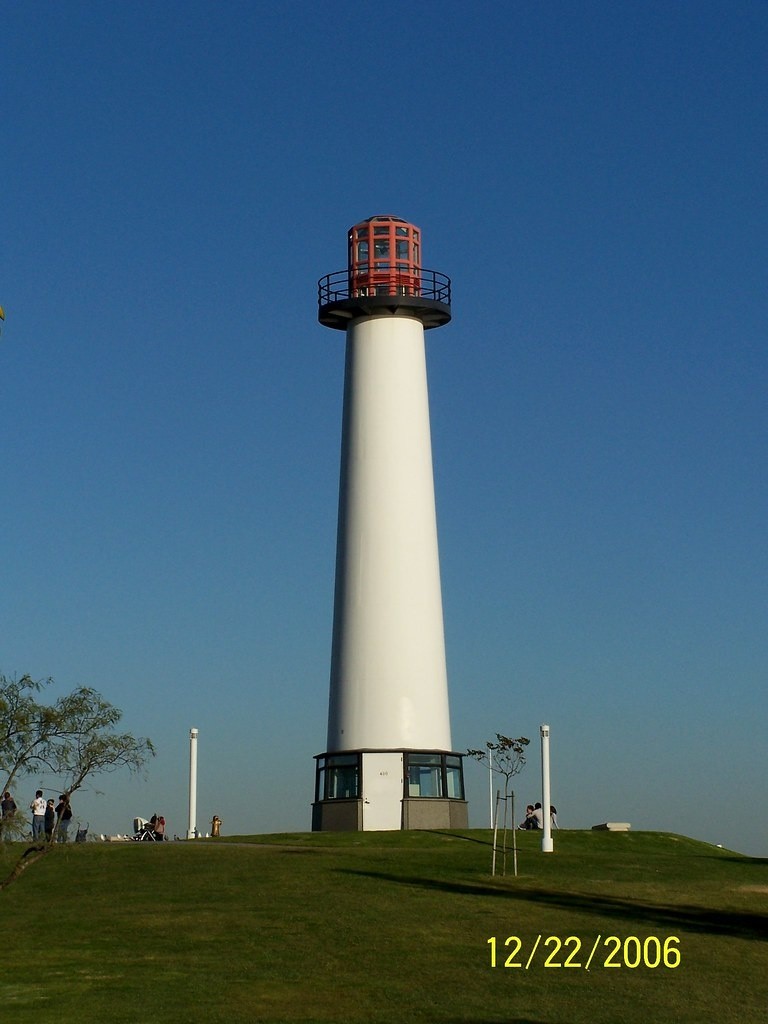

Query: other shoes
[{"left": 517, "top": 824, "right": 528, "bottom": 830}]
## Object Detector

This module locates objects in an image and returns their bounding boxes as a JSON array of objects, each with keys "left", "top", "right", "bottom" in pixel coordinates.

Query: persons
[
  {"left": 517, "top": 803, "right": 560, "bottom": 831},
  {"left": 150, "top": 813, "right": 165, "bottom": 842},
  {"left": 0, "top": 789, "right": 72, "bottom": 846}
]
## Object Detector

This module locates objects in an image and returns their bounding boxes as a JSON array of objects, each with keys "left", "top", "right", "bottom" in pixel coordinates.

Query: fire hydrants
[{"left": 210, "top": 814, "right": 222, "bottom": 838}]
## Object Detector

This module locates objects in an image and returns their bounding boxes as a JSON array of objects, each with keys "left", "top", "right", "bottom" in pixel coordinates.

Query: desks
[{"left": 592, "top": 822, "right": 630, "bottom": 831}]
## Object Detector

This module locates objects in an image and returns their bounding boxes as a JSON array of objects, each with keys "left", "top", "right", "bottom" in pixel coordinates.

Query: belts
[{"left": 34, "top": 815, "right": 46, "bottom": 817}]
